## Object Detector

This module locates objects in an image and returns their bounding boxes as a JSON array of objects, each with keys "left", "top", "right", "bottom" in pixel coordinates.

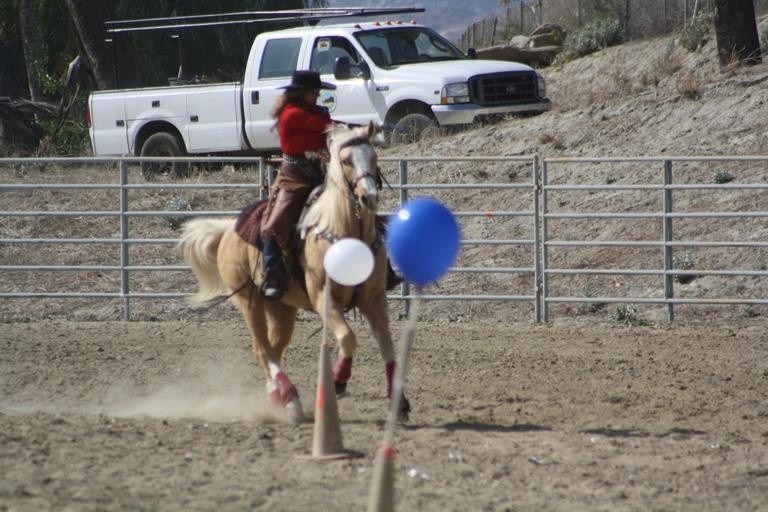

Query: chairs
[{"left": 363, "top": 47, "right": 389, "bottom": 67}]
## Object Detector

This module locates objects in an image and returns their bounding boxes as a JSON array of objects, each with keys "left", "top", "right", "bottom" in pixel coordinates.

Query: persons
[{"left": 259, "top": 71, "right": 331, "bottom": 299}]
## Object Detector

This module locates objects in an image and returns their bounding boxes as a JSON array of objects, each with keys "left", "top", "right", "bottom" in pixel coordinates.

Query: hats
[{"left": 275, "top": 71, "right": 336, "bottom": 91}]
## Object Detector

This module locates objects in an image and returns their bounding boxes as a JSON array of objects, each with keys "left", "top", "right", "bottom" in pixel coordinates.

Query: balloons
[
  {"left": 387, "top": 197, "right": 459, "bottom": 289},
  {"left": 324, "top": 238, "right": 374, "bottom": 288}
]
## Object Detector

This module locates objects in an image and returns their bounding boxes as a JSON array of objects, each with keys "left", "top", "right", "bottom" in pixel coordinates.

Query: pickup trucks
[{"left": 86, "top": 6, "right": 553, "bottom": 183}]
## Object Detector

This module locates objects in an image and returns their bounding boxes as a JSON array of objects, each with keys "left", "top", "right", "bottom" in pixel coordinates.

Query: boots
[
  {"left": 263, "top": 239, "right": 288, "bottom": 298},
  {"left": 387, "top": 258, "right": 404, "bottom": 290}
]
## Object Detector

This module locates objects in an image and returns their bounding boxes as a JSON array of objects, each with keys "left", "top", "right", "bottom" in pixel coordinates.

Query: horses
[{"left": 168, "top": 117, "right": 413, "bottom": 425}]
